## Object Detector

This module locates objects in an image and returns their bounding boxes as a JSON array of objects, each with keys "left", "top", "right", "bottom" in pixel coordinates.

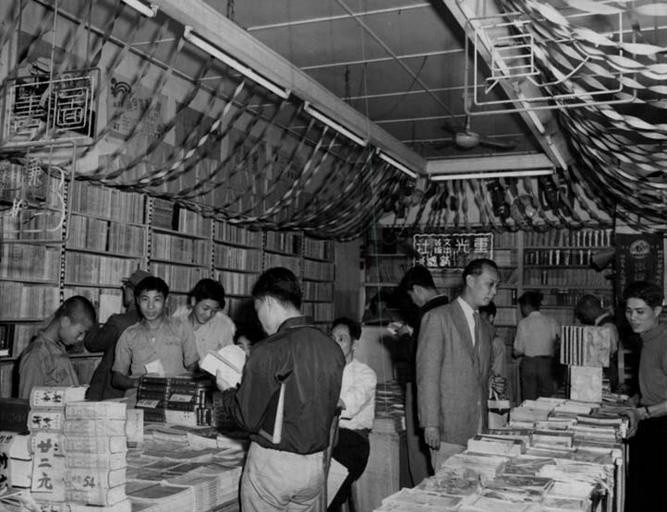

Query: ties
[{"left": 473, "top": 311, "right": 480, "bottom": 355}]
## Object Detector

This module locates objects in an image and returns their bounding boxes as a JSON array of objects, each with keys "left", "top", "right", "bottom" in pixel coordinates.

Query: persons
[{"left": 614, "top": 278, "right": 665, "bottom": 510}]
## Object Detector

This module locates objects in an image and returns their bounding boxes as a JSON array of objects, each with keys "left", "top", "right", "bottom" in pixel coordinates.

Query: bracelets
[{"left": 643, "top": 404, "right": 650, "bottom": 421}]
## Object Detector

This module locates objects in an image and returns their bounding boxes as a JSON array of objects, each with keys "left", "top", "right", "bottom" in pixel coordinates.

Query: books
[{"left": 0, "top": 154, "right": 637, "bottom": 512}]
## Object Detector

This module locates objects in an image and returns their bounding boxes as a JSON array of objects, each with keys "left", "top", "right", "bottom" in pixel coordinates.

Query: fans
[{"left": 432, "top": 91, "right": 519, "bottom": 154}]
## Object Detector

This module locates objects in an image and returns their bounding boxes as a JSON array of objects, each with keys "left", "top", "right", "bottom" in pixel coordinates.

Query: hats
[{"left": 122, "top": 270, "right": 150, "bottom": 286}]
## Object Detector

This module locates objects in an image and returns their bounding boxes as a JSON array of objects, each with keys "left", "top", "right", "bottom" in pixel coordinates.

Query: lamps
[{"left": 452, "top": 132, "right": 481, "bottom": 151}]
